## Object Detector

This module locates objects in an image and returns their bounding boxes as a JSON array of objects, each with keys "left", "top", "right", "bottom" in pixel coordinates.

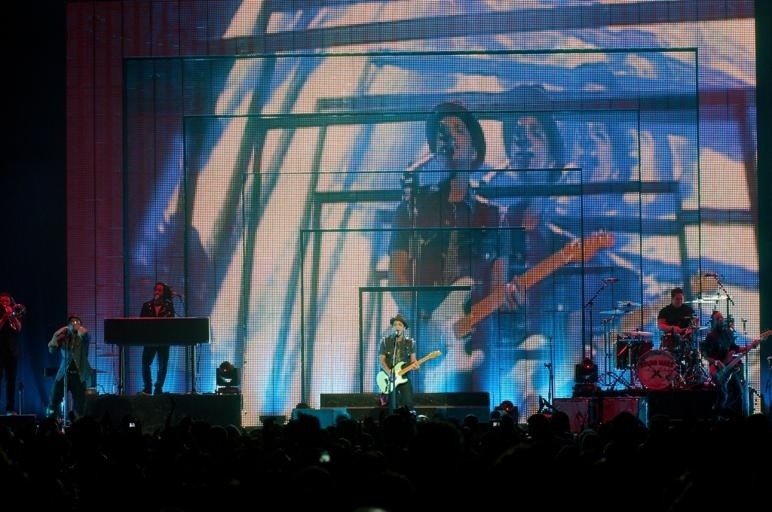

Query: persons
[
  {"left": 136, "top": 283, "right": 174, "bottom": 396},
  {"left": 700, "top": 310, "right": 745, "bottom": 410},
  {"left": 1, "top": 397, "right": 772, "bottom": 512},
  {"left": 47, "top": 316, "right": 91, "bottom": 418},
  {"left": 476, "top": 85, "right": 604, "bottom": 394},
  {"left": 388, "top": 99, "right": 527, "bottom": 392},
  {"left": 379, "top": 314, "right": 420, "bottom": 407},
  {"left": 658, "top": 287, "right": 699, "bottom": 352},
  {"left": 547, "top": 64, "right": 638, "bottom": 262},
  {"left": 0, "top": 291, "right": 27, "bottom": 415}
]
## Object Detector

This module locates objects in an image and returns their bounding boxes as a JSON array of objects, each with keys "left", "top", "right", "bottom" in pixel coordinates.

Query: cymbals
[
  {"left": 599, "top": 309, "right": 634, "bottom": 315},
  {"left": 617, "top": 300, "right": 641, "bottom": 307},
  {"left": 701, "top": 294, "right": 734, "bottom": 301},
  {"left": 686, "top": 299, "right": 714, "bottom": 305},
  {"left": 621, "top": 330, "right": 652, "bottom": 338}
]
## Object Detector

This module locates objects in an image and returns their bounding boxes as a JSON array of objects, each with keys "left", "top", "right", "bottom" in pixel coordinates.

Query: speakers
[
  {"left": 576, "top": 364, "right": 598, "bottom": 383},
  {"left": 216, "top": 368, "right": 240, "bottom": 385}
]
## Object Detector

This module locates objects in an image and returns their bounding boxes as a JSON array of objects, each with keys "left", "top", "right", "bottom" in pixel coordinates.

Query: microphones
[
  {"left": 403, "top": 150, "right": 439, "bottom": 178},
  {"left": 169, "top": 291, "right": 182, "bottom": 298},
  {"left": 395, "top": 330, "right": 399, "bottom": 338},
  {"left": 601, "top": 276, "right": 619, "bottom": 283},
  {"left": 543, "top": 159, "right": 577, "bottom": 217},
  {"left": 480, "top": 158, "right": 515, "bottom": 188}
]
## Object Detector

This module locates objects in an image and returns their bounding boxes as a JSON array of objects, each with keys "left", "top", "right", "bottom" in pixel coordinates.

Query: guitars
[
  {"left": 376, "top": 350, "right": 442, "bottom": 395},
  {"left": 489, "top": 272, "right": 719, "bottom": 424},
  {"left": 701, "top": 328, "right": 772, "bottom": 391},
  {"left": 381, "top": 228, "right": 616, "bottom": 393}
]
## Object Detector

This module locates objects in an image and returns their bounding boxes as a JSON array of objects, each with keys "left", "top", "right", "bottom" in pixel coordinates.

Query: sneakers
[{"left": 142, "top": 386, "right": 163, "bottom": 396}]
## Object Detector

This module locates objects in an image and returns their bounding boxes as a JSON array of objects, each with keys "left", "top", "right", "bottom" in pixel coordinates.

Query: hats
[
  {"left": 502, "top": 84, "right": 566, "bottom": 183},
  {"left": 391, "top": 313, "right": 408, "bottom": 330},
  {"left": 425, "top": 100, "right": 486, "bottom": 173}
]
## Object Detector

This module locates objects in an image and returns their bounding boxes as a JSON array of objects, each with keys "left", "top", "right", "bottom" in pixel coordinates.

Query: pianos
[{"left": 104, "top": 316, "right": 211, "bottom": 346}]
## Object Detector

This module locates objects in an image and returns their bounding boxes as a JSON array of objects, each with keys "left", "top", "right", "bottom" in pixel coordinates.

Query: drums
[
  {"left": 613, "top": 340, "right": 654, "bottom": 371},
  {"left": 635, "top": 347, "right": 680, "bottom": 392},
  {"left": 660, "top": 333, "right": 681, "bottom": 351}
]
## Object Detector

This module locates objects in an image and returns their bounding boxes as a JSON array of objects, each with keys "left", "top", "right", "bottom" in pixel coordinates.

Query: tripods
[
  {"left": 674, "top": 330, "right": 715, "bottom": 387},
  {"left": 594, "top": 322, "right": 647, "bottom": 391}
]
